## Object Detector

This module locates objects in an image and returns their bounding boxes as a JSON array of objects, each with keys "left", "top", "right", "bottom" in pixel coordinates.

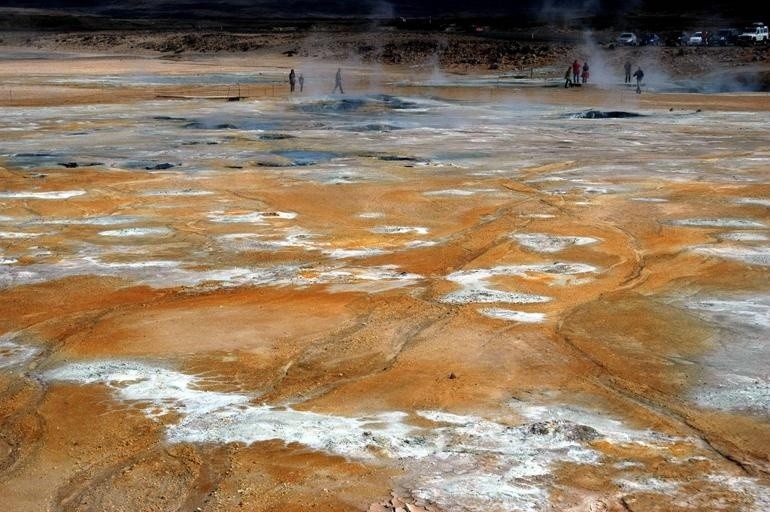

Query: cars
[{"left": 613, "top": 31, "right": 711, "bottom": 47}]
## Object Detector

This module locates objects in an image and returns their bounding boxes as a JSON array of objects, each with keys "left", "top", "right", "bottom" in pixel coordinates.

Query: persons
[
  {"left": 564, "top": 60, "right": 589, "bottom": 88},
  {"left": 700, "top": 29, "right": 706, "bottom": 47},
  {"left": 290, "top": 70, "right": 296, "bottom": 92},
  {"left": 299, "top": 74, "right": 304, "bottom": 91},
  {"left": 624, "top": 62, "right": 631, "bottom": 83},
  {"left": 332, "top": 68, "right": 345, "bottom": 93},
  {"left": 633, "top": 67, "right": 644, "bottom": 93},
  {"left": 707, "top": 31, "right": 713, "bottom": 48}
]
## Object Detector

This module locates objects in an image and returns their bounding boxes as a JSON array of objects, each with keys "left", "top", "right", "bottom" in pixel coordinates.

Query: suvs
[
  {"left": 712, "top": 29, "right": 737, "bottom": 46},
  {"left": 738, "top": 25, "right": 768, "bottom": 46}
]
